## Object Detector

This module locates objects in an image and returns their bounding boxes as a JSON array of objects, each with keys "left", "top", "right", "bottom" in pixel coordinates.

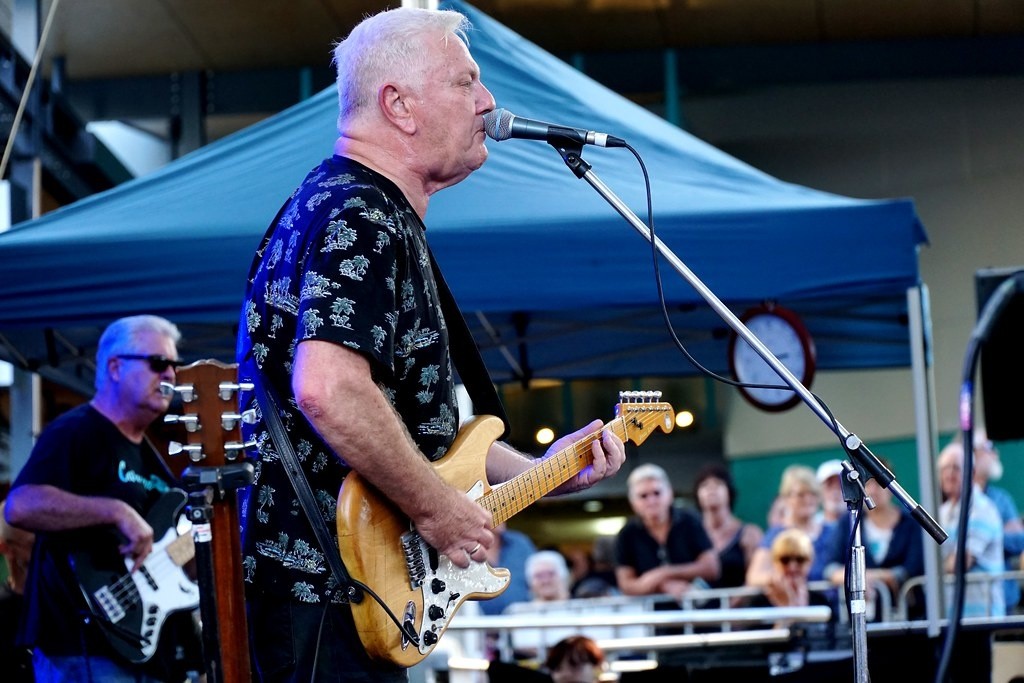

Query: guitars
[
  {"left": 158, "top": 359, "right": 260, "bottom": 681},
  {"left": 335, "top": 390, "right": 676, "bottom": 666},
  {"left": 69, "top": 513, "right": 210, "bottom": 665}
]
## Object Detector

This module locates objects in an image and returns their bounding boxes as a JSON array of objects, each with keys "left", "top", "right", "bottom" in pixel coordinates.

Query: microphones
[{"left": 485, "top": 108, "right": 626, "bottom": 148}]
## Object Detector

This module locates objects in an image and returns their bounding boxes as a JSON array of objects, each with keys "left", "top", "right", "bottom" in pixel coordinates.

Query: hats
[{"left": 817, "top": 459, "right": 844, "bottom": 484}]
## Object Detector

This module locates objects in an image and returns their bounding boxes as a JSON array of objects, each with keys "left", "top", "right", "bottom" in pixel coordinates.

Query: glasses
[
  {"left": 775, "top": 554, "right": 811, "bottom": 565},
  {"left": 629, "top": 489, "right": 664, "bottom": 500},
  {"left": 115, "top": 354, "right": 185, "bottom": 373}
]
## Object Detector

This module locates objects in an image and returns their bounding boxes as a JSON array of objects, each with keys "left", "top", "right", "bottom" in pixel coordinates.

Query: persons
[
  {"left": 4, "top": 315, "right": 196, "bottom": 683},
  {"left": 228, "top": 6, "right": 628, "bottom": 683},
  {"left": 479, "top": 431, "right": 1024, "bottom": 683}
]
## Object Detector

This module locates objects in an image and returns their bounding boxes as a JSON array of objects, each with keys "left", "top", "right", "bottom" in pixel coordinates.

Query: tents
[{"left": 0, "top": 0, "right": 942, "bottom": 638}]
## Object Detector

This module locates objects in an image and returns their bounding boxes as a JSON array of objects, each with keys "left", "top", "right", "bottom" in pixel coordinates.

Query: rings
[{"left": 470, "top": 543, "right": 481, "bottom": 554}]
